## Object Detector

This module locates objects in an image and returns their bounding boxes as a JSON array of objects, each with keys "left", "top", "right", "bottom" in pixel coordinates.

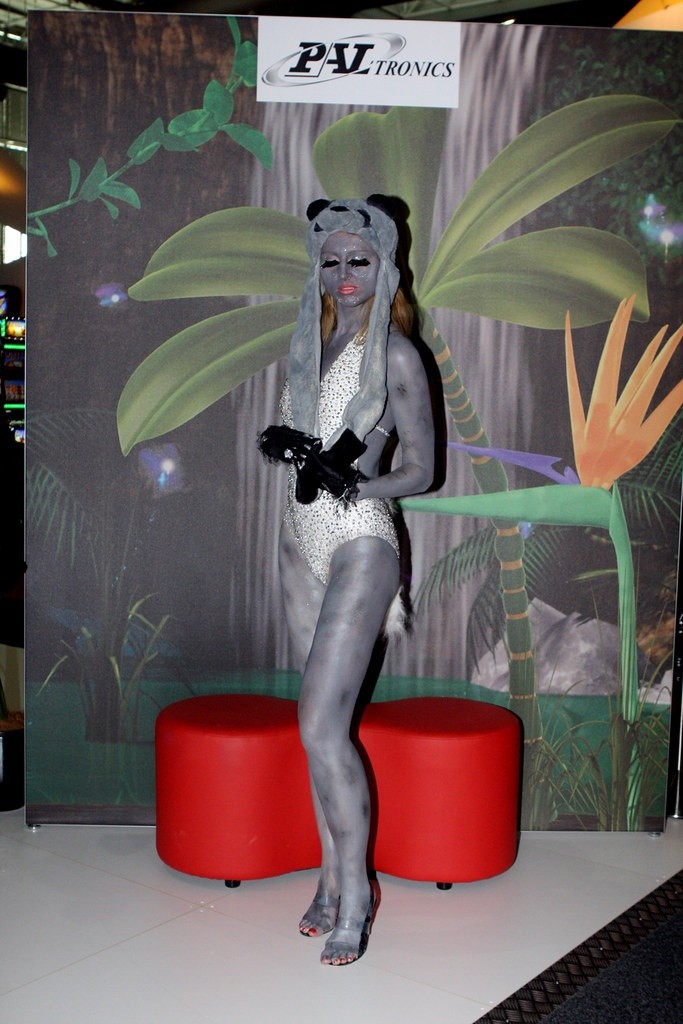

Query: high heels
[
  {"left": 299, "top": 891, "right": 340, "bottom": 937},
  {"left": 321, "top": 880, "right": 377, "bottom": 966}
]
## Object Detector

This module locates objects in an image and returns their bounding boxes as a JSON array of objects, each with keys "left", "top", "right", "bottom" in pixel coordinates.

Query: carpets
[{"left": 473, "top": 869, "right": 683, "bottom": 1024}]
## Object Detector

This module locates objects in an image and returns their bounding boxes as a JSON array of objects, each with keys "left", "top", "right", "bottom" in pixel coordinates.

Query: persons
[{"left": 277, "top": 199, "right": 435, "bottom": 967}]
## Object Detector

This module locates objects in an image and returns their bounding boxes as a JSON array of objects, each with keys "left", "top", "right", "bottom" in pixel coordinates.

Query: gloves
[
  {"left": 296, "top": 450, "right": 369, "bottom": 508},
  {"left": 259, "top": 424, "right": 322, "bottom": 477}
]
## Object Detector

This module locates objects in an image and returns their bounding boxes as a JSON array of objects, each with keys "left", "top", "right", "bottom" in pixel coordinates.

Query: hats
[{"left": 278, "top": 194, "right": 412, "bottom": 504}]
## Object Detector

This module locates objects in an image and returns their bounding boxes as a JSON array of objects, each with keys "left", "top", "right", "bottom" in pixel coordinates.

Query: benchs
[{"left": 154, "top": 694, "right": 522, "bottom": 890}]
